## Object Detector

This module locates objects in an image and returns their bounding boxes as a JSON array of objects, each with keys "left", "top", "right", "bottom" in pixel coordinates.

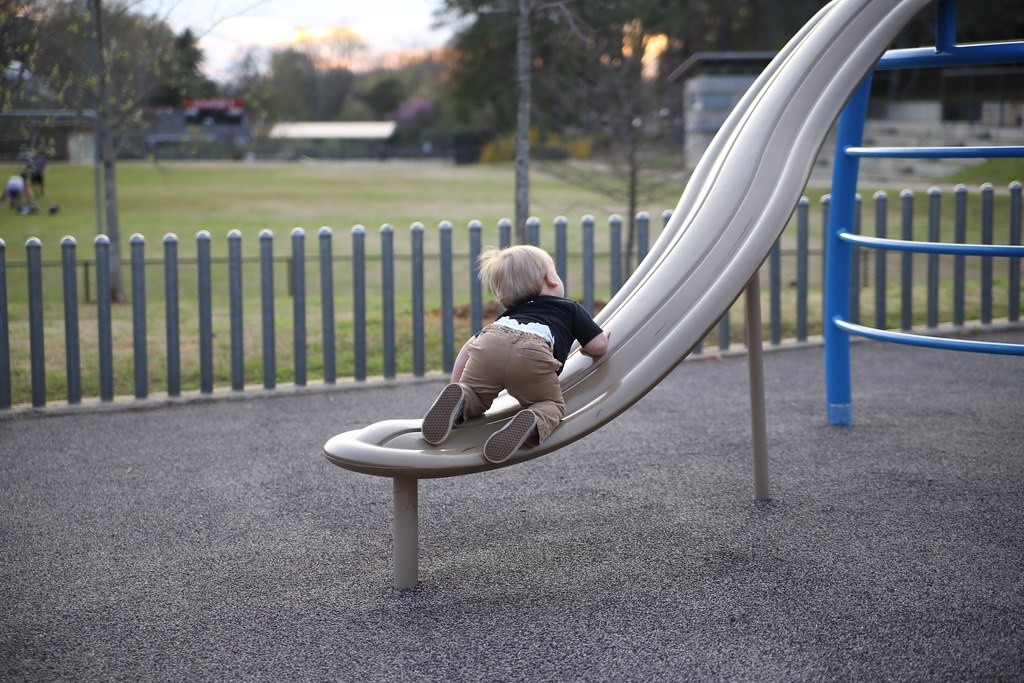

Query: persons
[
  {"left": 0, "top": 150, "right": 48, "bottom": 217},
  {"left": 421, "top": 244, "right": 610, "bottom": 464}
]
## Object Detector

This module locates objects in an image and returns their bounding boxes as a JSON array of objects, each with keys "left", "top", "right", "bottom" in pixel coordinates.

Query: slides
[{"left": 320, "top": 0, "right": 934, "bottom": 481}]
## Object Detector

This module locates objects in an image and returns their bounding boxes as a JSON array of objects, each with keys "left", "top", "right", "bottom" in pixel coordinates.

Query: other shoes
[
  {"left": 483, "top": 408, "right": 538, "bottom": 464},
  {"left": 421, "top": 382, "right": 466, "bottom": 446}
]
